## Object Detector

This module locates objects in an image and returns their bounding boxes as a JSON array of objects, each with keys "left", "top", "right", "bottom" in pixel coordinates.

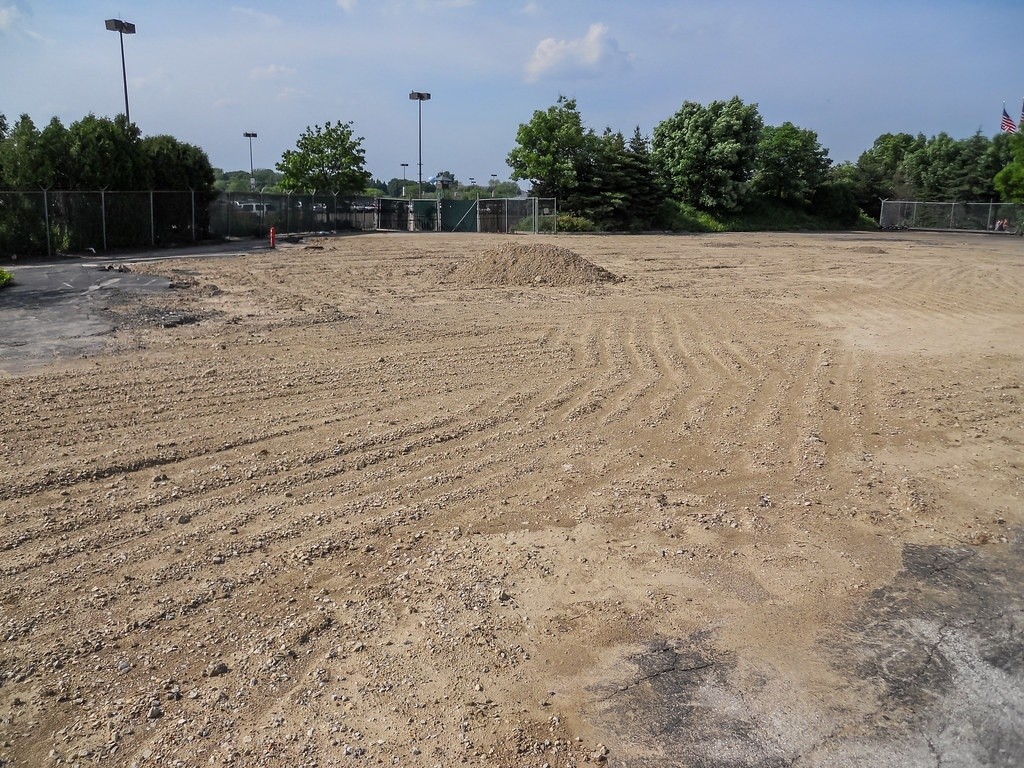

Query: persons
[{"left": 995, "top": 218, "right": 1008, "bottom": 231}]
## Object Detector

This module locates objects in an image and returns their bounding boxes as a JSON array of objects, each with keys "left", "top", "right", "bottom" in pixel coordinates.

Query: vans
[{"left": 226, "top": 199, "right": 503, "bottom": 220}]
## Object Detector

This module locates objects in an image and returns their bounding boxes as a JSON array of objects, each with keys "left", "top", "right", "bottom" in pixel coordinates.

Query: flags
[
  {"left": 1001, "top": 108, "right": 1016, "bottom": 134},
  {"left": 1020, "top": 104, "right": 1024, "bottom": 122}
]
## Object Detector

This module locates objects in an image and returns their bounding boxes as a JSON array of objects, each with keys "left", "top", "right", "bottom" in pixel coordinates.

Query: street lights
[
  {"left": 469, "top": 178, "right": 474, "bottom": 185},
  {"left": 409, "top": 90, "right": 431, "bottom": 199},
  {"left": 491, "top": 174, "right": 497, "bottom": 187},
  {"left": 243, "top": 131, "right": 257, "bottom": 177},
  {"left": 400, "top": 163, "right": 408, "bottom": 196},
  {"left": 472, "top": 181, "right": 476, "bottom": 185},
  {"left": 104, "top": 19, "right": 136, "bottom": 135}
]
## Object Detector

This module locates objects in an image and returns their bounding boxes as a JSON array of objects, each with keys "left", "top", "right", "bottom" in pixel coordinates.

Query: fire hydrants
[{"left": 269, "top": 225, "right": 276, "bottom": 249}]
